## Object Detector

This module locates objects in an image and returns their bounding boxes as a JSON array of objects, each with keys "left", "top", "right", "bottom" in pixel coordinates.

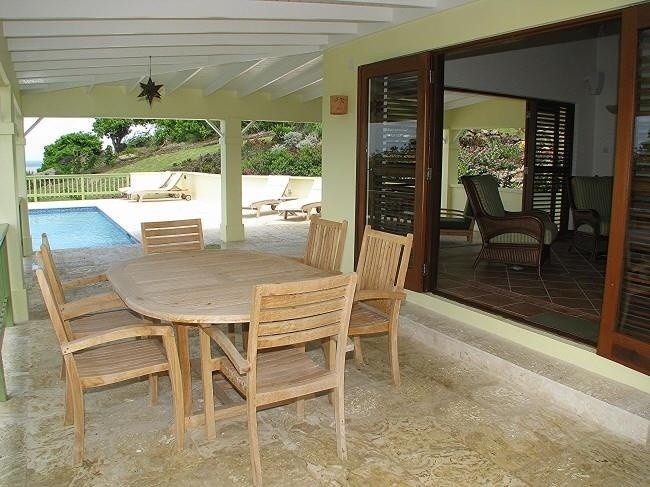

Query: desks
[{"left": 104, "top": 248, "right": 352, "bottom": 457}]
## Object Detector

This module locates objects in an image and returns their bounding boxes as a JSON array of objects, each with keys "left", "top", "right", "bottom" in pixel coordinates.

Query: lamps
[{"left": 329, "top": 93, "right": 349, "bottom": 116}]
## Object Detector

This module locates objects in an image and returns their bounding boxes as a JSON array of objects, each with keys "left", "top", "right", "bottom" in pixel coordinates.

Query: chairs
[
  {"left": 28, "top": 234, "right": 190, "bottom": 469},
  {"left": 241, "top": 173, "right": 293, "bottom": 219},
  {"left": 37, "top": 228, "right": 153, "bottom": 380},
  {"left": 274, "top": 173, "right": 323, "bottom": 221},
  {"left": 240, "top": 212, "right": 350, "bottom": 359},
  {"left": 138, "top": 218, "right": 236, "bottom": 350},
  {"left": 130, "top": 168, "right": 193, "bottom": 204},
  {"left": 199, "top": 269, "right": 360, "bottom": 487},
  {"left": 316, "top": 218, "right": 416, "bottom": 397},
  {"left": 439, "top": 195, "right": 477, "bottom": 243},
  {"left": 119, "top": 169, "right": 173, "bottom": 204},
  {"left": 567, "top": 174, "right": 616, "bottom": 266},
  {"left": 456, "top": 172, "right": 558, "bottom": 276}
]
